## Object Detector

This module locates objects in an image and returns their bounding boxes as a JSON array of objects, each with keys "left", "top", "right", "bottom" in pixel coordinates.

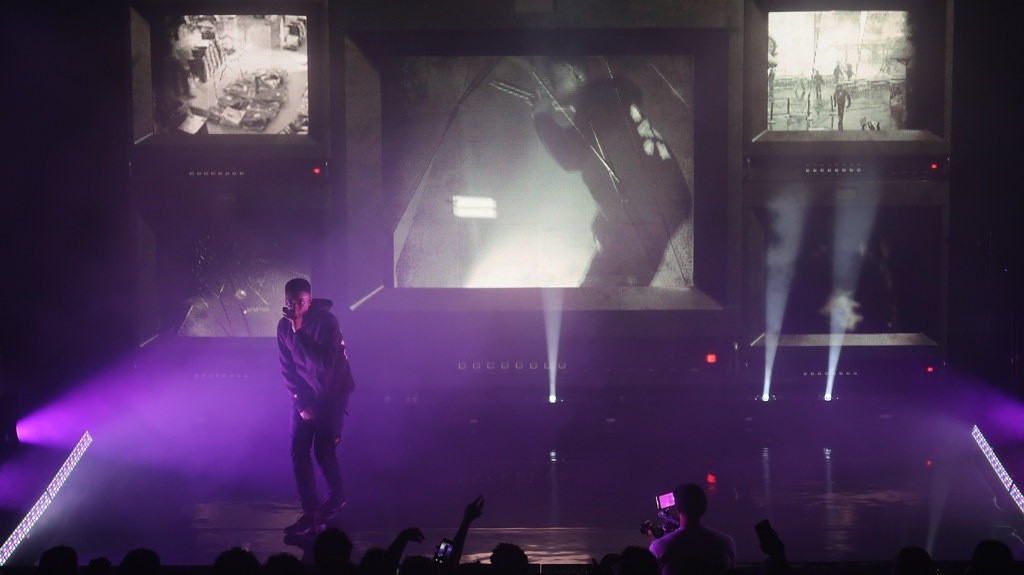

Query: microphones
[{"left": 283, "top": 307, "right": 295, "bottom": 318}]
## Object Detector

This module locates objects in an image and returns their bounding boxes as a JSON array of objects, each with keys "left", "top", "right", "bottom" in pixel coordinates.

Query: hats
[{"left": 674, "top": 482, "right": 708, "bottom": 517}]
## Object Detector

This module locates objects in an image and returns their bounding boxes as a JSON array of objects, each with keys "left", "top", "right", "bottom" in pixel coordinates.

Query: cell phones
[
  {"left": 434, "top": 538, "right": 454, "bottom": 566},
  {"left": 754, "top": 519, "right": 774, "bottom": 547}
]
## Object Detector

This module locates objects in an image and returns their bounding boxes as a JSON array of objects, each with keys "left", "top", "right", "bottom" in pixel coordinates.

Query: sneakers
[{"left": 283, "top": 514, "right": 314, "bottom": 535}]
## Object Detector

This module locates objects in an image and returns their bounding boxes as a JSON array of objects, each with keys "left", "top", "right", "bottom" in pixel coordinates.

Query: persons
[
  {"left": 39, "top": 483, "right": 1014, "bottom": 575},
  {"left": 813, "top": 62, "right": 852, "bottom": 124},
  {"left": 768, "top": 68, "right": 775, "bottom": 90},
  {"left": 526, "top": 54, "right": 692, "bottom": 287},
  {"left": 277, "top": 278, "right": 356, "bottom": 535}
]
